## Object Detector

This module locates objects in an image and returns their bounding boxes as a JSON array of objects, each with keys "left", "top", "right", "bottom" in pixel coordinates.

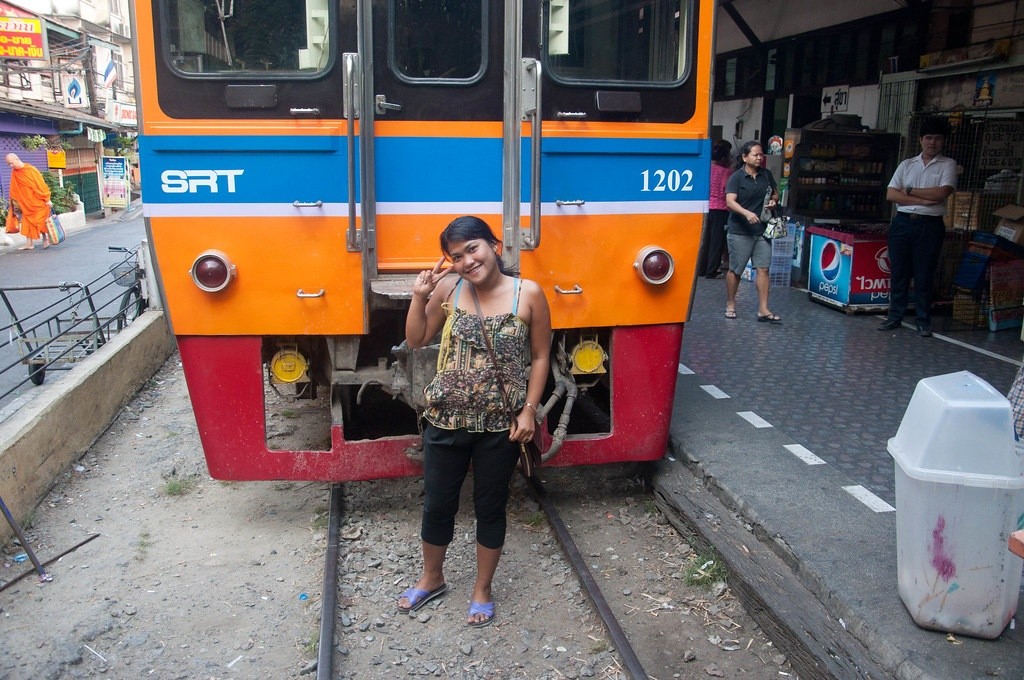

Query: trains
[{"left": 126, "top": 0, "right": 716, "bottom": 481}]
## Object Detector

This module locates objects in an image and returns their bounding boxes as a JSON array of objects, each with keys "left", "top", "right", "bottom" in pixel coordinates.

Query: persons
[
  {"left": 698, "top": 139, "right": 734, "bottom": 280},
  {"left": 725, "top": 141, "right": 781, "bottom": 321},
  {"left": 877, "top": 117, "right": 957, "bottom": 338},
  {"left": 5, "top": 152, "right": 53, "bottom": 250},
  {"left": 396, "top": 215, "right": 553, "bottom": 629}
]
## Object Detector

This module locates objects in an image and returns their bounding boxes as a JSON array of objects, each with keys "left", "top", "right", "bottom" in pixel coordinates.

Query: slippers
[
  {"left": 467, "top": 601, "right": 495, "bottom": 627},
  {"left": 725, "top": 306, "right": 737, "bottom": 318},
  {"left": 756, "top": 312, "right": 783, "bottom": 323},
  {"left": 397, "top": 583, "right": 447, "bottom": 614}
]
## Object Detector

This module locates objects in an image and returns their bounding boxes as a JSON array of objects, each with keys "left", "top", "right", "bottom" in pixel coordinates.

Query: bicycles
[{"left": 106, "top": 244, "right": 150, "bottom": 322}]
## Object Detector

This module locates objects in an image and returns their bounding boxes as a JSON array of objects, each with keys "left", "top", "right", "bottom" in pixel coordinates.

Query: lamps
[{"left": 916, "top": 52, "right": 1005, "bottom": 73}]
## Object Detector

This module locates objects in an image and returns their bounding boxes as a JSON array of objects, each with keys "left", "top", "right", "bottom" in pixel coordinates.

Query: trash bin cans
[{"left": 887, "top": 371, "right": 1023, "bottom": 640}]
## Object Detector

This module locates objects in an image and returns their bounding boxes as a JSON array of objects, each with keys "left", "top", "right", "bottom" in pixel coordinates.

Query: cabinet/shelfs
[{"left": 783, "top": 127, "right": 900, "bottom": 282}]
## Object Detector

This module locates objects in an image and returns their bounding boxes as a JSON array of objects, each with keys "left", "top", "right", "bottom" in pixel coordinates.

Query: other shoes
[
  {"left": 43, "top": 242, "right": 49, "bottom": 250},
  {"left": 877, "top": 320, "right": 901, "bottom": 330},
  {"left": 17, "top": 245, "right": 35, "bottom": 250},
  {"left": 707, "top": 272, "right": 726, "bottom": 279},
  {"left": 918, "top": 323, "right": 931, "bottom": 336}
]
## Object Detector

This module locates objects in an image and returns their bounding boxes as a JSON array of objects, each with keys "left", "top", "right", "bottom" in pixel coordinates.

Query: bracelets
[
  {"left": 525, "top": 403, "right": 538, "bottom": 413},
  {"left": 771, "top": 197, "right": 778, "bottom": 204}
]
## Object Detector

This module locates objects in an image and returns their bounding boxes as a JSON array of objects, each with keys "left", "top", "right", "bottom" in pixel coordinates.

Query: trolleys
[{"left": 1, "top": 283, "right": 121, "bottom": 384}]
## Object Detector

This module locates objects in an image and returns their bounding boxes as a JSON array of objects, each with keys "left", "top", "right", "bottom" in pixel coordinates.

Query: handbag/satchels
[
  {"left": 45, "top": 206, "right": 65, "bottom": 245},
  {"left": 5, "top": 204, "right": 20, "bottom": 233},
  {"left": 519, "top": 417, "right": 542, "bottom": 480},
  {"left": 764, "top": 204, "right": 786, "bottom": 239}
]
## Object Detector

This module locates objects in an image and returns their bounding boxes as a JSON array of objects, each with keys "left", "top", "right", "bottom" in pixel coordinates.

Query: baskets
[{"left": 110, "top": 261, "right": 139, "bottom": 287}]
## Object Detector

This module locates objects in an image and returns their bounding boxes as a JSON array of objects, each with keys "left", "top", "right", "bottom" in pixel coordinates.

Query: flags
[{"left": 103, "top": 53, "right": 117, "bottom": 88}]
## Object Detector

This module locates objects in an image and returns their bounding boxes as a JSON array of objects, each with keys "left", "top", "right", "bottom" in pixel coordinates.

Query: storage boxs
[
  {"left": 941, "top": 191, "right": 1024, "bottom": 331},
  {"left": 769, "top": 221, "right": 795, "bottom": 287}
]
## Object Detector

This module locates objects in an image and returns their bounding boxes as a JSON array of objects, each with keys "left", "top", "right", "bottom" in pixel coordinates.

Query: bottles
[{"left": 799, "top": 162, "right": 883, "bottom": 212}]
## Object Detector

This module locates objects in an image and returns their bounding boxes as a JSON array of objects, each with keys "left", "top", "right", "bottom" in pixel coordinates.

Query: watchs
[{"left": 907, "top": 187, "right": 912, "bottom": 193}]
